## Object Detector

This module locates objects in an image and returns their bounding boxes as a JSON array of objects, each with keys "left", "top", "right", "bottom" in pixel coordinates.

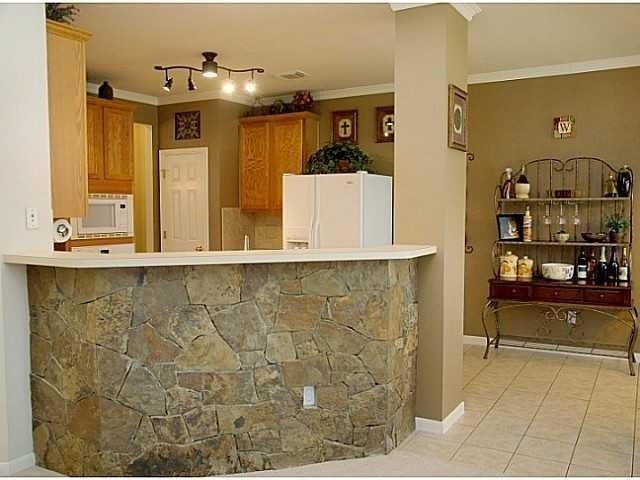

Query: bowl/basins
[
  {"left": 541, "top": 263, "right": 575, "bottom": 282},
  {"left": 579, "top": 233, "right": 609, "bottom": 241},
  {"left": 552, "top": 232, "right": 570, "bottom": 243}
]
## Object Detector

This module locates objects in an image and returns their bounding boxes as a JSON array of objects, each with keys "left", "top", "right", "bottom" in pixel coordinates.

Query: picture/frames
[
  {"left": 497, "top": 213, "right": 525, "bottom": 241},
  {"left": 448, "top": 83, "right": 468, "bottom": 152},
  {"left": 376, "top": 106, "right": 395, "bottom": 142},
  {"left": 331, "top": 108, "right": 359, "bottom": 145}
]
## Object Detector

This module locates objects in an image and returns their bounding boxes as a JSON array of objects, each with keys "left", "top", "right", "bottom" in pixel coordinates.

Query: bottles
[
  {"left": 515, "top": 163, "right": 530, "bottom": 198},
  {"left": 522, "top": 205, "right": 533, "bottom": 243},
  {"left": 602, "top": 165, "right": 633, "bottom": 197},
  {"left": 577, "top": 247, "right": 629, "bottom": 282},
  {"left": 98, "top": 80, "right": 113, "bottom": 100},
  {"left": 500, "top": 251, "right": 534, "bottom": 282}
]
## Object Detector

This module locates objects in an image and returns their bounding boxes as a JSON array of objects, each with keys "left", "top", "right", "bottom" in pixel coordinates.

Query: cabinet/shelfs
[
  {"left": 86, "top": 95, "right": 136, "bottom": 194},
  {"left": 481, "top": 158, "right": 639, "bottom": 376},
  {"left": 238, "top": 111, "right": 320, "bottom": 211}
]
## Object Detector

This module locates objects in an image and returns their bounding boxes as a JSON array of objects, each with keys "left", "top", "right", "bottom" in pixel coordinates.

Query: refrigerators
[{"left": 282, "top": 171, "right": 392, "bottom": 249}]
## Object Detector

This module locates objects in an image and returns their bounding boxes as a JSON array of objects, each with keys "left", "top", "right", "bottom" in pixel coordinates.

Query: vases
[{"left": 98, "top": 80, "right": 113, "bottom": 98}]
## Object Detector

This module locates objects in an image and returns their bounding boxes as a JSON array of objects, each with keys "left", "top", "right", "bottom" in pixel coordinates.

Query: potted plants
[
  {"left": 305, "top": 140, "right": 368, "bottom": 173},
  {"left": 604, "top": 213, "right": 628, "bottom": 243}
]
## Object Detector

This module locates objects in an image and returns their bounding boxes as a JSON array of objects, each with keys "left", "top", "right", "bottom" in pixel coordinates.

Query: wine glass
[{"left": 542, "top": 203, "right": 581, "bottom": 225}]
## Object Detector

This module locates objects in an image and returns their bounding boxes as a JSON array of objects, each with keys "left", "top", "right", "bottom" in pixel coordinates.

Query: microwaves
[{"left": 70, "top": 194, "right": 135, "bottom": 240}]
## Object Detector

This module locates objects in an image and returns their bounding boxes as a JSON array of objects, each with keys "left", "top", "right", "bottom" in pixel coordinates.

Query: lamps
[{"left": 153, "top": 51, "right": 264, "bottom": 93}]
